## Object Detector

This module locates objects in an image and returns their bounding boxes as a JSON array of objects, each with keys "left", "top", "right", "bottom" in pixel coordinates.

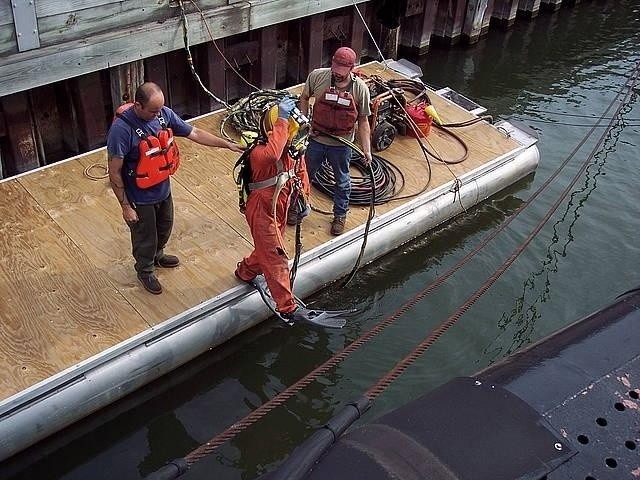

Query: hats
[{"left": 331, "top": 46, "right": 357, "bottom": 77}]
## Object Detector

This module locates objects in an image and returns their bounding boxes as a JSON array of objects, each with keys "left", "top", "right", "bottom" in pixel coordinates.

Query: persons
[
  {"left": 285, "top": 45, "right": 374, "bottom": 236},
  {"left": 106, "top": 81, "right": 249, "bottom": 295},
  {"left": 233, "top": 96, "right": 359, "bottom": 331}
]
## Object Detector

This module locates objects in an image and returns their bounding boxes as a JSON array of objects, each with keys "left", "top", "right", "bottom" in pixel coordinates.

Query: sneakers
[
  {"left": 154, "top": 253, "right": 179, "bottom": 268},
  {"left": 137, "top": 271, "right": 163, "bottom": 294},
  {"left": 330, "top": 215, "right": 346, "bottom": 235}
]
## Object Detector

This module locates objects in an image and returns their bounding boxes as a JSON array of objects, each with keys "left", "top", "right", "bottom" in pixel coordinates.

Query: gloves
[{"left": 277, "top": 95, "right": 297, "bottom": 120}]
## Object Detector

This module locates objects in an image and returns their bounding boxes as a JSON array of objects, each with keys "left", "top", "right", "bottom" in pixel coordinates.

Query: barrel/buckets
[{"left": 407, "top": 103, "right": 431, "bottom": 138}]
